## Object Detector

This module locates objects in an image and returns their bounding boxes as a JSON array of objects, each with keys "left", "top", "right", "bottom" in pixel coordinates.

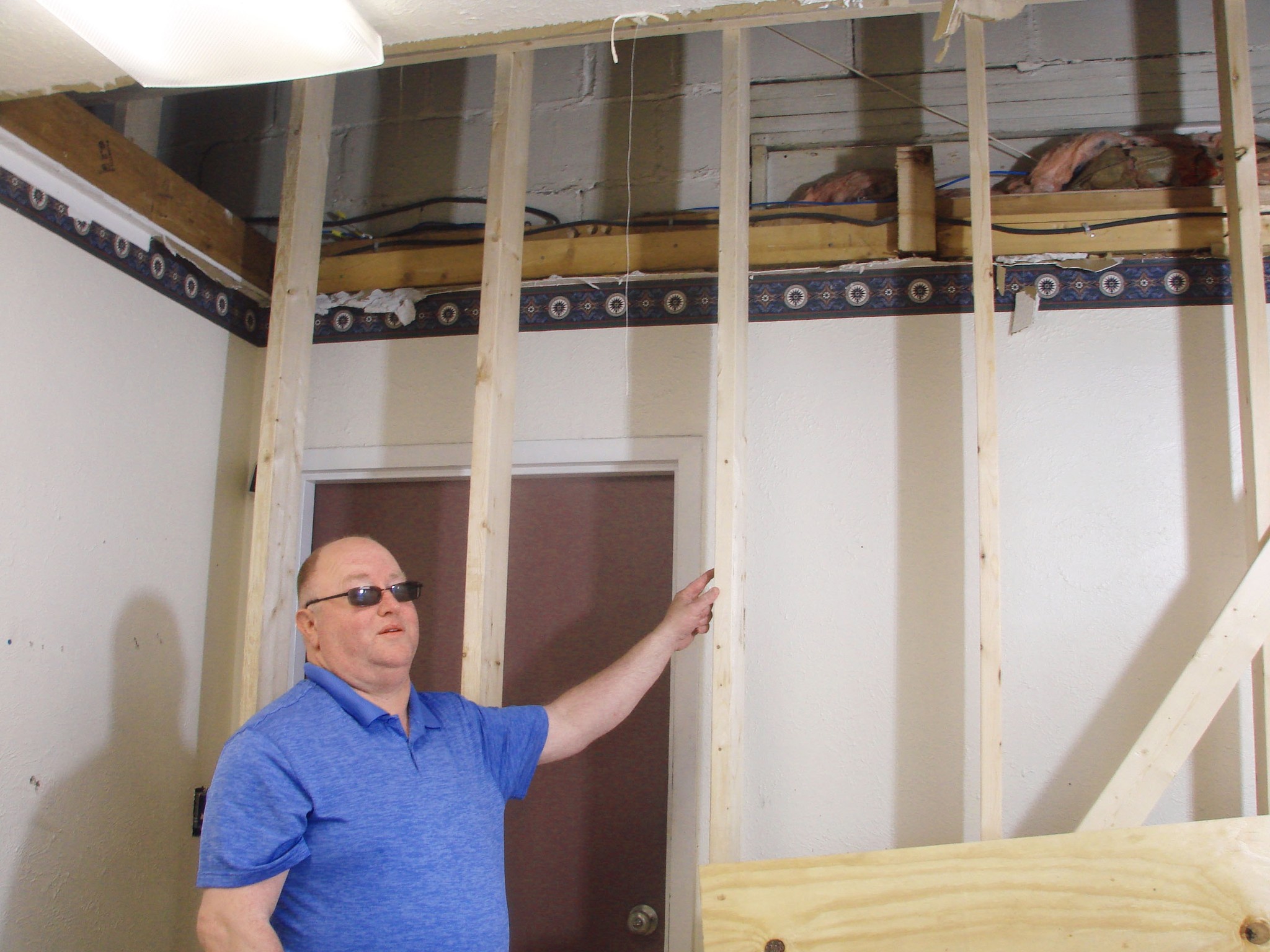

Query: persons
[{"left": 192, "top": 538, "right": 719, "bottom": 952}]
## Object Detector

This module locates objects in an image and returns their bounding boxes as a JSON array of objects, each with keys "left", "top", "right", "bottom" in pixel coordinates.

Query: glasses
[{"left": 305, "top": 581, "right": 423, "bottom": 609}]
